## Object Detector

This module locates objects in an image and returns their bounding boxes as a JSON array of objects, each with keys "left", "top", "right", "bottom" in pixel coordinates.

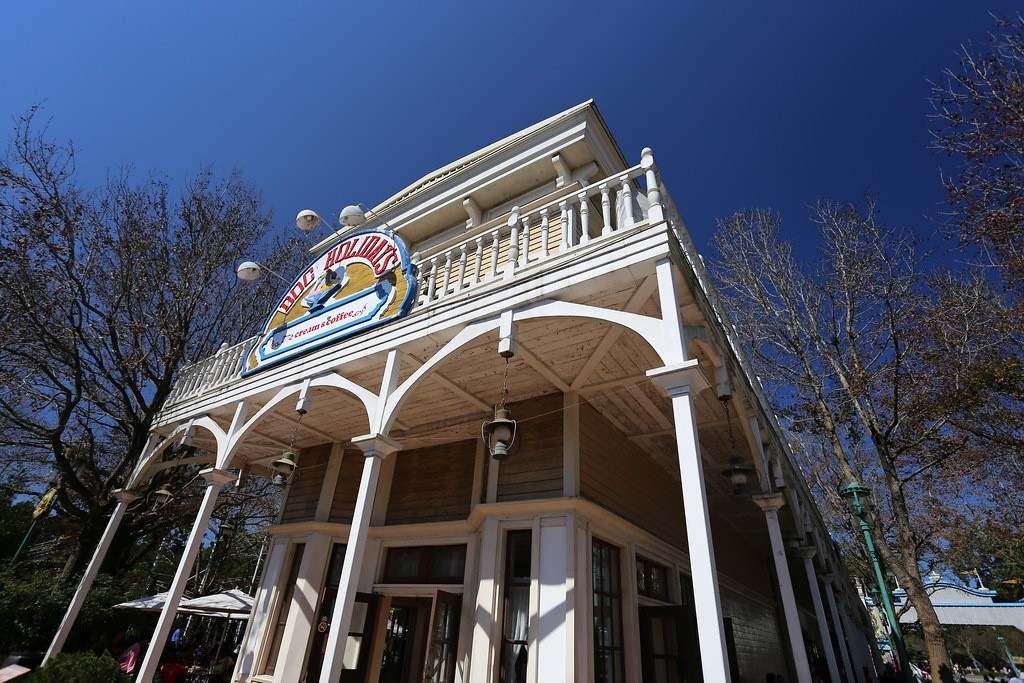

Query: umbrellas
[{"left": 110, "top": 589, "right": 254, "bottom": 665}]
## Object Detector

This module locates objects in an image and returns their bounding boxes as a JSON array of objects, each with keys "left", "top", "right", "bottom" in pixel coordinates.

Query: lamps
[
  {"left": 720, "top": 400, "right": 762, "bottom": 503},
  {"left": 338, "top": 203, "right": 399, "bottom": 237},
  {"left": 236, "top": 261, "right": 292, "bottom": 285},
  {"left": 295, "top": 210, "right": 343, "bottom": 239},
  {"left": 783, "top": 527, "right": 808, "bottom": 556},
  {"left": 481, "top": 358, "right": 521, "bottom": 460},
  {"left": 267, "top": 414, "right": 304, "bottom": 491},
  {"left": 147, "top": 449, "right": 176, "bottom": 521}
]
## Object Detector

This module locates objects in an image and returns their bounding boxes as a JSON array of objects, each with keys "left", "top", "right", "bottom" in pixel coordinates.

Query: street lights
[
  {"left": 997, "top": 635, "right": 1020, "bottom": 679},
  {"left": 838, "top": 478, "right": 914, "bottom": 683}
]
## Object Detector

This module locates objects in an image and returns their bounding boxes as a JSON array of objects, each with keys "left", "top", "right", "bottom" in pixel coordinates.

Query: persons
[
  {"left": 765, "top": 661, "right": 1024, "bottom": 683},
  {"left": 97, "top": 623, "right": 236, "bottom": 683}
]
